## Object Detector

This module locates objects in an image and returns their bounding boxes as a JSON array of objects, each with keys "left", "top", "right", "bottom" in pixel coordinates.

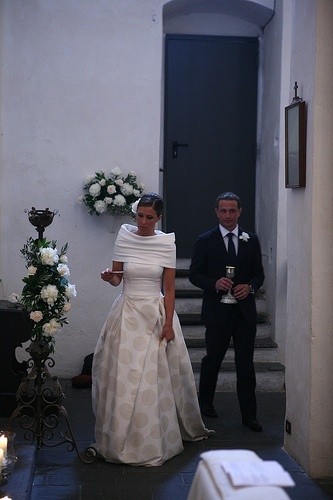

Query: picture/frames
[{"left": 285, "top": 101, "right": 306, "bottom": 188}]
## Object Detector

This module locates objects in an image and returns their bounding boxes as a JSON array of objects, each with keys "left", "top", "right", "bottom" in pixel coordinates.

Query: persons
[
  {"left": 91, "top": 192, "right": 214, "bottom": 468},
  {"left": 187, "top": 192, "right": 265, "bottom": 434}
]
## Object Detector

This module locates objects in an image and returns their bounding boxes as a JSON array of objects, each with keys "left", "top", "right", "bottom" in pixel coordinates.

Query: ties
[{"left": 226, "top": 233, "right": 237, "bottom": 266}]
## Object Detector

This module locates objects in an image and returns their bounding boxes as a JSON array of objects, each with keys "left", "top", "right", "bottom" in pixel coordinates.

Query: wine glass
[{"left": 220, "top": 265, "right": 235, "bottom": 300}]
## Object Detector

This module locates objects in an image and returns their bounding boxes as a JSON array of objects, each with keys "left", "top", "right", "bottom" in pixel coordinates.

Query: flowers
[
  {"left": 8, "top": 236, "right": 77, "bottom": 344},
  {"left": 82, "top": 168, "right": 145, "bottom": 221},
  {"left": 239, "top": 232, "right": 250, "bottom": 242}
]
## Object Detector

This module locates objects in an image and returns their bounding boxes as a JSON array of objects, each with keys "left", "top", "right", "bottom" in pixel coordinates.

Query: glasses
[{"left": 217, "top": 209, "right": 239, "bottom": 214}]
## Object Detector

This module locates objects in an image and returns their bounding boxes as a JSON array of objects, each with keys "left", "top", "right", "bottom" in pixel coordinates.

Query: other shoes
[
  {"left": 243, "top": 417, "right": 263, "bottom": 430},
  {"left": 198, "top": 398, "right": 218, "bottom": 417}
]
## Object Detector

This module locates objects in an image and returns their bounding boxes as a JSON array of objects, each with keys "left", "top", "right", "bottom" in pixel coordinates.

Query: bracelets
[{"left": 249, "top": 284, "right": 253, "bottom": 292}]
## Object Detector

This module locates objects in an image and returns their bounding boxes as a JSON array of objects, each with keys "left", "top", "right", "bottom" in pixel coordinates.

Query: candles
[{"left": 0, "top": 434, "right": 8, "bottom": 467}]
